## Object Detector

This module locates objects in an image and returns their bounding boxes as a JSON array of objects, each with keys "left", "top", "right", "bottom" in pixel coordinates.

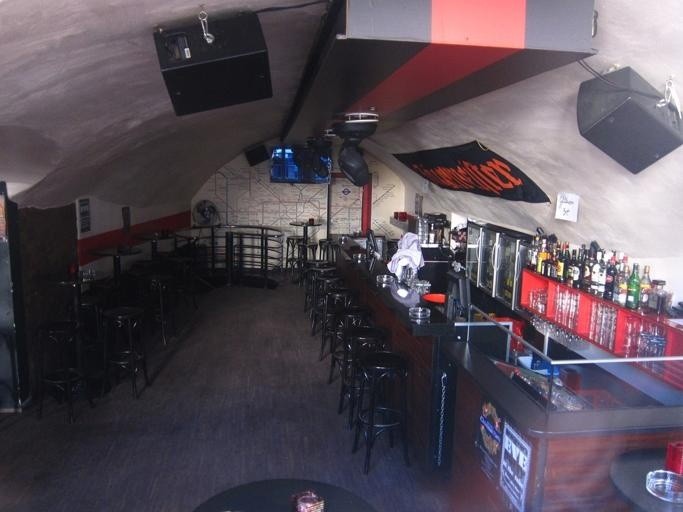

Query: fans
[{"left": 191, "top": 199, "right": 221, "bottom": 227}]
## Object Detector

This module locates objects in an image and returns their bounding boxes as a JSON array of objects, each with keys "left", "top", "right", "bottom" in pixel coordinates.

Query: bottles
[{"left": 526, "top": 235, "right": 672, "bottom": 319}]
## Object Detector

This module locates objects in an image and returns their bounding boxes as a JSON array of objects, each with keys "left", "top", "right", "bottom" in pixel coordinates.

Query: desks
[
  {"left": 289, "top": 221, "right": 322, "bottom": 286},
  {"left": 608, "top": 447, "right": 683, "bottom": 512},
  {"left": 134, "top": 230, "right": 175, "bottom": 260},
  {"left": 87, "top": 243, "right": 143, "bottom": 275},
  {"left": 192, "top": 477, "right": 378, "bottom": 512}
]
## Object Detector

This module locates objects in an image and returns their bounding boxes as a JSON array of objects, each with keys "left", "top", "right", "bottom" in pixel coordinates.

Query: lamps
[{"left": 291, "top": 122, "right": 377, "bottom": 187}]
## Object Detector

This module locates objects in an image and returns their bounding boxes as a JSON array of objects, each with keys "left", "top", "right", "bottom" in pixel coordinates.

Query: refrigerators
[{"left": 466, "top": 223, "right": 535, "bottom": 317}]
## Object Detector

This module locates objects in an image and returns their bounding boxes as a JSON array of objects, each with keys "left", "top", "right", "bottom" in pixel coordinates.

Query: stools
[
  {"left": 34, "top": 255, "right": 199, "bottom": 425},
  {"left": 284, "top": 236, "right": 411, "bottom": 475}
]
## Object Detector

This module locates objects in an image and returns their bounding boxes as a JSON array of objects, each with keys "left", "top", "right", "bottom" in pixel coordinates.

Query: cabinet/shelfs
[
  {"left": 519, "top": 268, "right": 683, "bottom": 391},
  {"left": 464, "top": 218, "right": 536, "bottom": 323}
]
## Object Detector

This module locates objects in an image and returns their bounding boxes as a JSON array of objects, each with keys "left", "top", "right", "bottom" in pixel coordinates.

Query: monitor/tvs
[
  {"left": 445, "top": 261, "right": 472, "bottom": 309},
  {"left": 366, "top": 227, "right": 379, "bottom": 251},
  {"left": 269, "top": 144, "right": 332, "bottom": 184}
]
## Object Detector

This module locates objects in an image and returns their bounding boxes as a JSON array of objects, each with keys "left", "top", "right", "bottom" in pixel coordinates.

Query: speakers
[
  {"left": 152, "top": 13, "right": 274, "bottom": 117},
  {"left": 577, "top": 67, "right": 683, "bottom": 175},
  {"left": 245, "top": 145, "right": 269, "bottom": 167}
]
